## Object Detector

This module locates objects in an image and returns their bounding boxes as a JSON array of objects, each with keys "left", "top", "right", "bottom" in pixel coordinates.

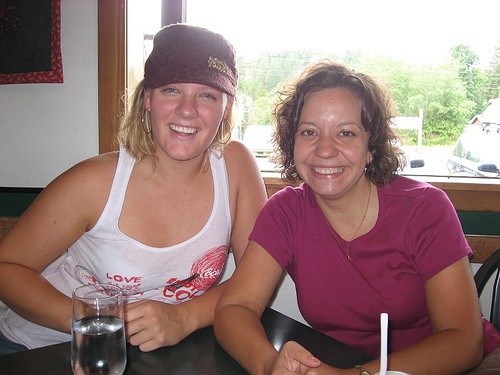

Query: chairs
[{"left": 389, "top": 108, "right": 424, "bottom": 155}]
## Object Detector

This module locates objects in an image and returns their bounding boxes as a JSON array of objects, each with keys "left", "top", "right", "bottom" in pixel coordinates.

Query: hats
[{"left": 144, "top": 23, "right": 237, "bottom": 96}]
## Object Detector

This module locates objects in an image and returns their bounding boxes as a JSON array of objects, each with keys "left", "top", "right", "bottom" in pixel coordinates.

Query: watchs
[{"left": 354, "top": 364, "right": 370, "bottom": 375}]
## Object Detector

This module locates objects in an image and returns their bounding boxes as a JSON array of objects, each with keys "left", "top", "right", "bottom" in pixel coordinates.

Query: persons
[
  {"left": 210, "top": 58, "right": 500, "bottom": 375},
  {"left": 0, "top": 24, "right": 268, "bottom": 356}
]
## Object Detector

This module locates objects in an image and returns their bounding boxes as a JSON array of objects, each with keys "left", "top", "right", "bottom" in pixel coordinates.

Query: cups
[
  {"left": 72, "top": 282, "right": 126, "bottom": 375},
  {"left": 374, "top": 370, "right": 410, "bottom": 375}
]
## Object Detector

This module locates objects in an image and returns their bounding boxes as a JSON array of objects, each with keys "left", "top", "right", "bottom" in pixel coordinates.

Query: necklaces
[{"left": 317, "top": 179, "right": 371, "bottom": 260}]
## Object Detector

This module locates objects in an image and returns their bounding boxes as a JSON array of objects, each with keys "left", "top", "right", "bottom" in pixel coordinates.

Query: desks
[{"left": 0, "top": 308, "right": 374, "bottom": 375}]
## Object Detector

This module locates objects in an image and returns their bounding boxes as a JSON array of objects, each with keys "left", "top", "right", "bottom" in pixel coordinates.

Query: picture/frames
[{"left": 0, "top": 0, "right": 64, "bottom": 85}]
